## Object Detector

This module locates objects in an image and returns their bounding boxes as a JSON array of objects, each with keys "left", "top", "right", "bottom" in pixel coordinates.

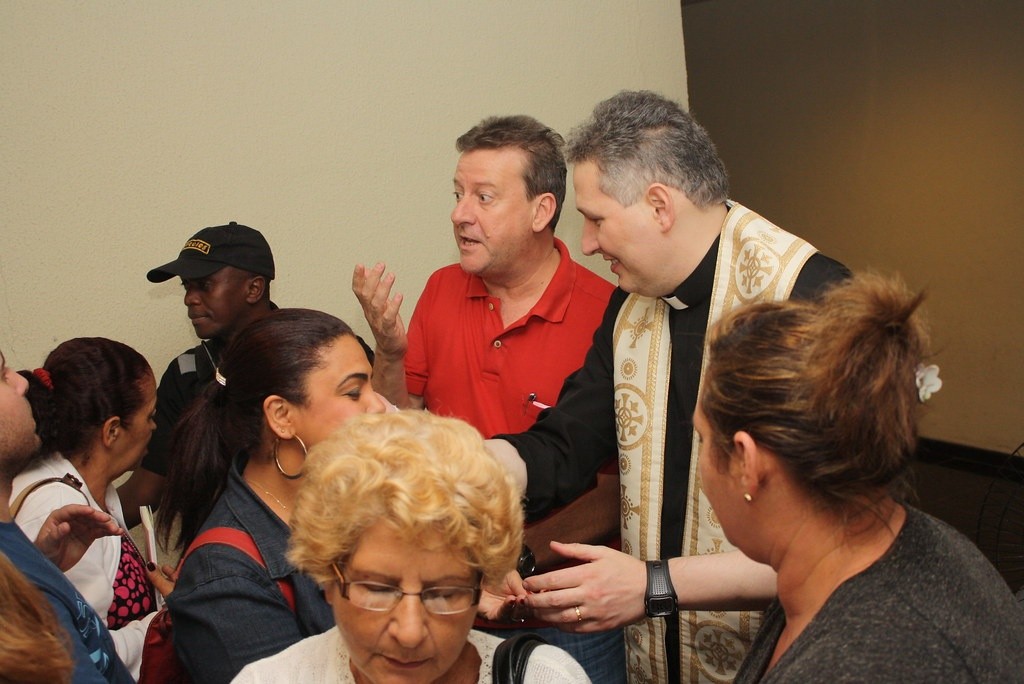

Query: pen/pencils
[{"left": 522, "top": 392, "right": 537, "bottom": 416}]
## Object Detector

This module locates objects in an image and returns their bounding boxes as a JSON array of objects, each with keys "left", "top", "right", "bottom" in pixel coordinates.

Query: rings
[
  {"left": 510, "top": 617, "right": 524, "bottom": 622},
  {"left": 575, "top": 606, "right": 582, "bottom": 622}
]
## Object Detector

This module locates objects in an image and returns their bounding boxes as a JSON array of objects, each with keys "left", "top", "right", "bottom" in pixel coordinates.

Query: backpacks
[{"left": 137, "top": 528, "right": 295, "bottom": 684}]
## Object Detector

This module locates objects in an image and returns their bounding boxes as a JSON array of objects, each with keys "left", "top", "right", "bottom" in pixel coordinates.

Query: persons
[
  {"left": 231, "top": 409, "right": 594, "bottom": 684},
  {"left": 0, "top": 553, "right": 72, "bottom": 684},
  {"left": 487, "top": 85, "right": 856, "bottom": 684},
  {"left": 353, "top": 119, "right": 627, "bottom": 684},
  {"left": 165, "top": 308, "right": 387, "bottom": 684},
  {"left": 0, "top": 350, "right": 139, "bottom": 684},
  {"left": 118, "top": 221, "right": 280, "bottom": 547},
  {"left": 693, "top": 272, "right": 1024, "bottom": 684},
  {"left": 10, "top": 336, "right": 175, "bottom": 684}
]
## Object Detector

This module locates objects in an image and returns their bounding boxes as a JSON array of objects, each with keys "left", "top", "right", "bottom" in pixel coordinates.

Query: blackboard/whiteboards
[{"left": 0, "top": 0, "right": 690, "bottom": 389}]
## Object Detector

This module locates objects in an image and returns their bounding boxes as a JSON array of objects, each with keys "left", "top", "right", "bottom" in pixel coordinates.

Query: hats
[{"left": 146, "top": 221, "right": 275, "bottom": 284}]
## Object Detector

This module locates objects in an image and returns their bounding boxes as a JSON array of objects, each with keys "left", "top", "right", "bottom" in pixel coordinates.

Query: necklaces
[
  {"left": 124, "top": 532, "right": 146, "bottom": 568},
  {"left": 247, "top": 479, "right": 287, "bottom": 510}
]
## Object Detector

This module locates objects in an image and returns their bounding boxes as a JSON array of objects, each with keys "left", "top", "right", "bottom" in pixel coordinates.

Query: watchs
[
  {"left": 644, "top": 559, "right": 678, "bottom": 618},
  {"left": 516, "top": 544, "right": 536, "bottom": 578}
]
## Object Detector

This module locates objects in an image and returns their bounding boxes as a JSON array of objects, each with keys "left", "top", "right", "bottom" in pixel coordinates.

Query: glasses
[{"left": 331, "top": 564, "right": 483, "bottom": 614}]
